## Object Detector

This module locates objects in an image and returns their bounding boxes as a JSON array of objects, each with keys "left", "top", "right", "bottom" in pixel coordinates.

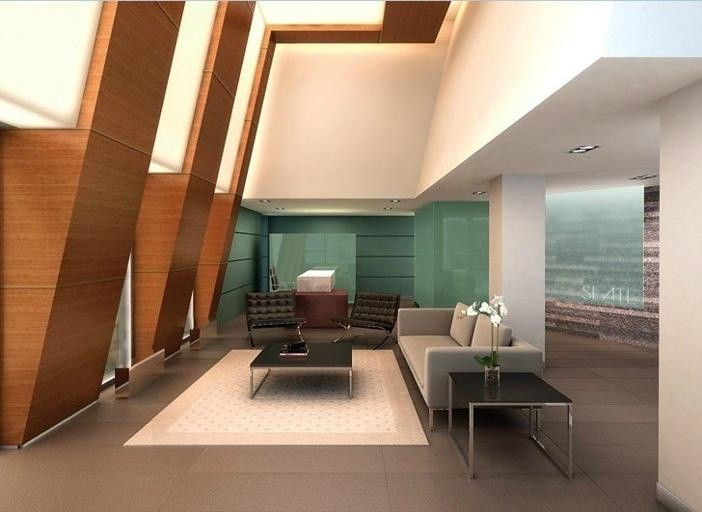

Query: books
[{"left": 279, "top": 348, "right": 310, "bottom": 356}]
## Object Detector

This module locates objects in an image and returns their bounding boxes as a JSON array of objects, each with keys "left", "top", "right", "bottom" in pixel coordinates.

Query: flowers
[{"left": 455, "top": 294, "right": 508, "bottom": 373}]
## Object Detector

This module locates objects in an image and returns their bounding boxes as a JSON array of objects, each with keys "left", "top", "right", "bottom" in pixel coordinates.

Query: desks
[
  {"left": 445, "top": 367, "right": 575, "bottom": 482},
  {"left": 277, "top": 290, "right": 350, "bottom": 329}
]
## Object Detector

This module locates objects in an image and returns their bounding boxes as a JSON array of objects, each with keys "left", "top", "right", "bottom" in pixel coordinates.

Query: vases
[{"left": 482, "top": 364, "right": 501, "bottom": 389}]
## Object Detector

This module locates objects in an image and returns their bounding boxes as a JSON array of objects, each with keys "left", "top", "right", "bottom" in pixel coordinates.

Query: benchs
[
  {"left": 113, "top": 347, "right": 166, "bottom": 399},
  {"left": 189, "top": 319, "right": 219, "bottom": 350}
]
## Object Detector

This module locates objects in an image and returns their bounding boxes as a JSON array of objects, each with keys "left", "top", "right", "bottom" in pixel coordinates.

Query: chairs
[
  {"left": 243, "top": 287, "right": 308, "bottom": 347},
  {"left": 268, "top": 264, "right": 296, "bottom": 292},
  {"left": 326, "top": 291, "right": 401, "bottom": 351}
]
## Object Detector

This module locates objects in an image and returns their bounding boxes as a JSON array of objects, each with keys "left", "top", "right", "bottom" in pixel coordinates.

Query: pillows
[
  {"left": 447, "top": 302, "right": 479, "bottom": 346},
  {"left": 470, "top": 313, "right": 513, "bottom": 347}
]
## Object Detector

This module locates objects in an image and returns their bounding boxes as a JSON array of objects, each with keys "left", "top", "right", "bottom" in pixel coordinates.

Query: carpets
[{"left": 118, "top": 346, "right": 430, "bottom": 451}]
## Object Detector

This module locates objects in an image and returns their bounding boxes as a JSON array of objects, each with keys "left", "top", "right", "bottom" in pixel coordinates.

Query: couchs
[{"left": 395, "top": 304, "right": 543, "bottom": 431}]
